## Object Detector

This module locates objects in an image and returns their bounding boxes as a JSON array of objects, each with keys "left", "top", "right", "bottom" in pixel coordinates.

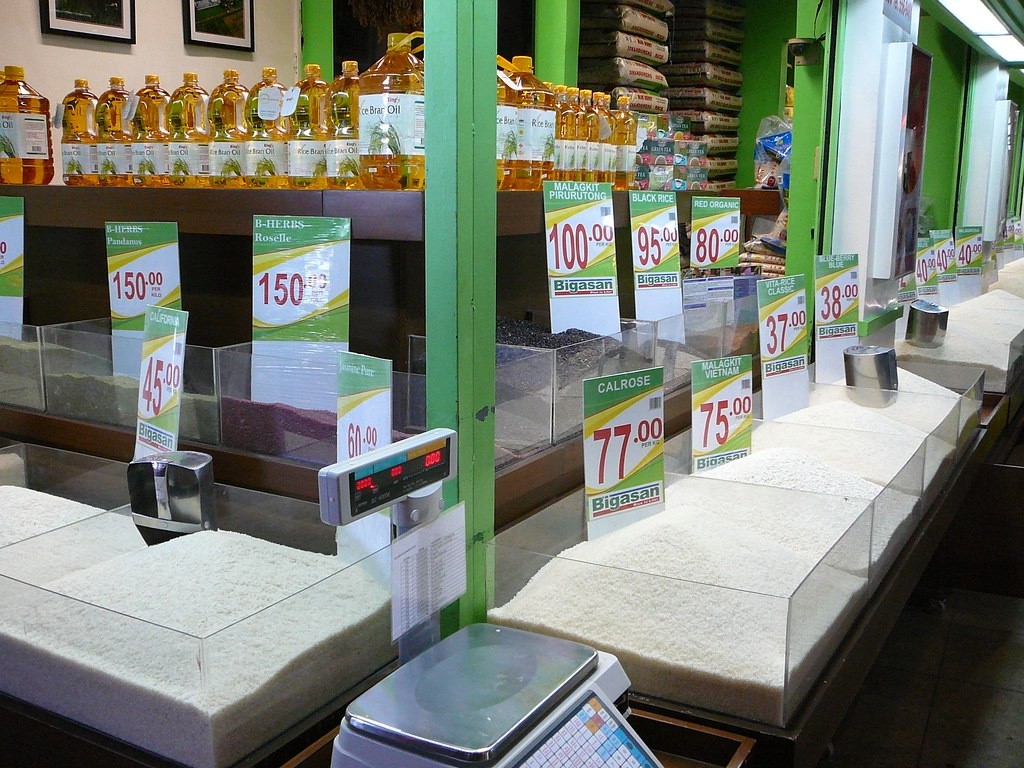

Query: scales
[{"left": 319, "top": 427, "right": 666, "bottom": 768}]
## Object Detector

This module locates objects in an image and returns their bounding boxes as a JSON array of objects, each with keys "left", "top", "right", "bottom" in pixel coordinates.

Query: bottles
[
  {"left": 61, "top": 79, "right": 100, "bottom": 185},
  {"left": 497, "top": 55, "right": 615, "bottom": 190},
  {"left": 0, "top": 65, "right": 55, "bottom": 185},
  {"left": 244, "top": 66, "right": 288, "bottom": 190},
  {"left": 615, "top": 96, "right": 639, "bottom": 190},
  {"left": 132, "top": 74, "right": 170, "bottom": 176},
  {"left": 167, "top": 73, "right": 211, "bottom": 176},
  {"left": 95, "top": 77, "right": 132, "bottom": 185},
  {"left": 208, "top": 69, "right": 250, "bottom": 189},
  {"left": 359, "top": 32, "right": 425, "bottom": 190},
  {"left": 325, "top": 61, "right": 359, "bottom": 190},
  {"left": 288, "top": 63, "right": 330, "bottom": 190}
]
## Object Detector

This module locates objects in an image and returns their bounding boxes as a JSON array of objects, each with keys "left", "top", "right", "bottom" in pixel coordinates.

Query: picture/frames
[
  {"left": 182, "top": 0, "right": 255, "bottom": 52},
  {"left": 38, "top": 0, "right": 137, "bottom": 45}
]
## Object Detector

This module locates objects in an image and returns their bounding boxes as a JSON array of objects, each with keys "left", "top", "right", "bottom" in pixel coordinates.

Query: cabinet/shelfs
[{"left": 0, "top": 186, "right": 785, "bottom": 532}]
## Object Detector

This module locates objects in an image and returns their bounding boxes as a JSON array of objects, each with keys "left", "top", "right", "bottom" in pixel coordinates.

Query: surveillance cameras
[{"left": 788, "top": 43, "right": 809, "bottom": 57}]
